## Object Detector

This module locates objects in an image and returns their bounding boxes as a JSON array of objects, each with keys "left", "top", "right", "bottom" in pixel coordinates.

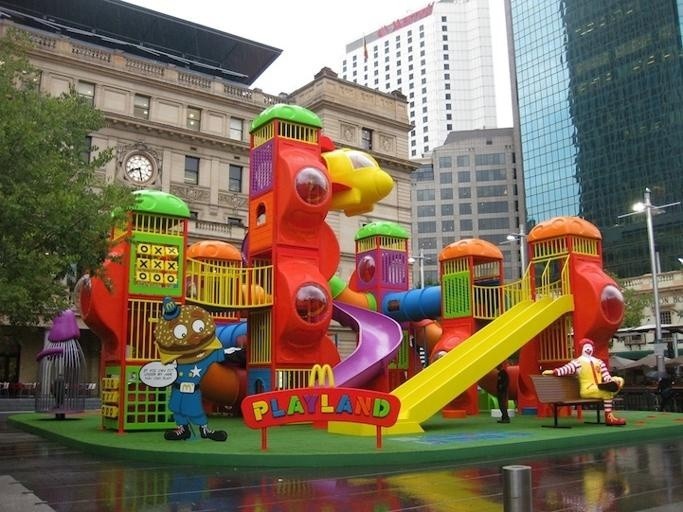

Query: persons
[
  {"left": 655, "top": 376, "right": 676, "bottom": 412},
  {"left": 495, "top": 363, "right": 512, "bottom": 425},
  {"left": 540, "top": 340, "right": 628, "bottom": 428},
  {"left": 148, "top": 293, "right": 243, "bottom": 443}
]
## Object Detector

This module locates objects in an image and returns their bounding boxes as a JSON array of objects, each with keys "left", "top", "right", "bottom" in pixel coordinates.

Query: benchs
[{"left": 528, "top": 374, "right": 626, "bottom": 430}]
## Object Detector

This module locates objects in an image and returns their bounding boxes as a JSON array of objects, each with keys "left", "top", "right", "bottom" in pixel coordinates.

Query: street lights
[
  {"left": 507, "top": 224, "right": 526, "bottom": 279},
  {"left": 407, "top": 245, "right": 424, "bottom": 290},
  {"left": 632, "top": 189, "right": 666, "bottom": 385}
]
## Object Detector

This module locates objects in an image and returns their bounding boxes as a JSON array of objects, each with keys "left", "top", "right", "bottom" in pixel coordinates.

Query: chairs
[{"left": 0, "top": 381, "right": 97, "bottom": 398}]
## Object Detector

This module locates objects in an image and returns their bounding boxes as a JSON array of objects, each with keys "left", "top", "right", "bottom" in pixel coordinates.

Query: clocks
[{"left": 125, "top": 154, "right": 153, "bottom": 184}]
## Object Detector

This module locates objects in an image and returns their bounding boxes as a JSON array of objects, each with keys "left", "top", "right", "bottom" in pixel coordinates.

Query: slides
[
  {"left": 302, "top": 300, "right": 403, "bottom": 389},
  {"left": 414, "top": 316, "right": 536, "bottom": 415},
  {"left": 327, "top": 291, "right": 576, "bottom": 436}
]
